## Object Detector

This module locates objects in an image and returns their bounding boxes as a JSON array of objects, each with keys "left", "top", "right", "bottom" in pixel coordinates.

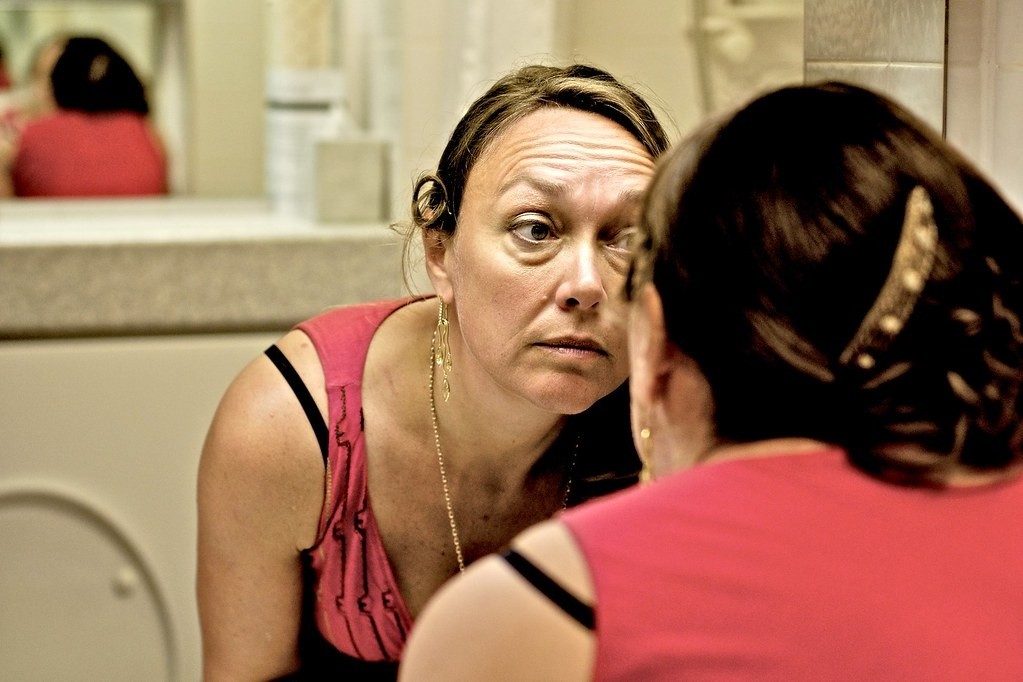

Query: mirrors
[
  {"left": 1, "top": 0, "right": 262, "bottom": 205},
  {"left": 0, "top": 1, "right": 944, "bottom": 679}
]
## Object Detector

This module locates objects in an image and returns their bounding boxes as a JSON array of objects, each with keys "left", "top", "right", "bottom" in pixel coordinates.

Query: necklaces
[{"left": 429, "top": 331, "right": 580, "bottom": 576}]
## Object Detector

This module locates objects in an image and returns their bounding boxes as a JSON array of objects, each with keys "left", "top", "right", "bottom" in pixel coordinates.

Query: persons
[
  {"left": 0, "top": 36, "right": 171, "bottom": 197},
  {"left": 399, "top": 82, "right": 1023, "bottom": 682},
  {"left": 193, "top": 66, "right": 671, "bottom": 682}
]
